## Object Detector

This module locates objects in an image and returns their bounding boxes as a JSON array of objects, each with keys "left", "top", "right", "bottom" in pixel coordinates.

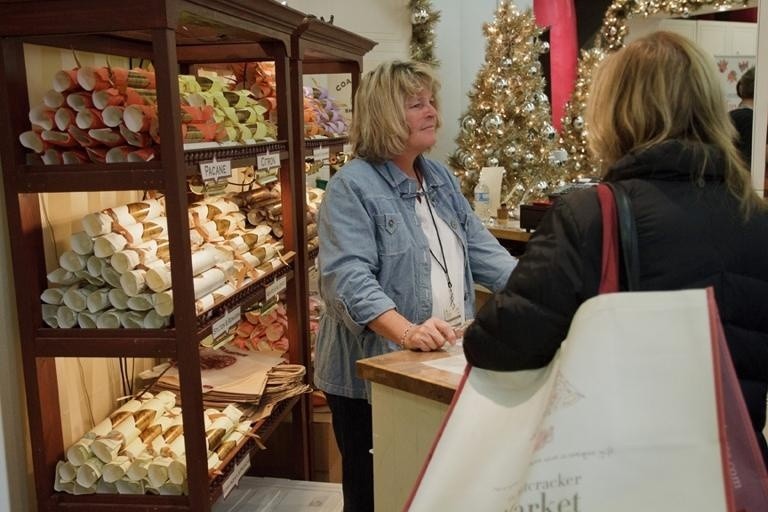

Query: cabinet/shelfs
[{"left": 2, "top": 1, "right": 379, "bottom": 512}]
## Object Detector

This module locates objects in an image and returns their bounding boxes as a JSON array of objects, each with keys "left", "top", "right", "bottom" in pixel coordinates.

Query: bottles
[{"left": 473, "top": 178, "right": 490, "bottom": 223}]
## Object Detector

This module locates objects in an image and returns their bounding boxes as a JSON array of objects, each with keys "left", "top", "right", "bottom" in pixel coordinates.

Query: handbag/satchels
[{"left": 403, "top": 183, "right": 768, "bottom": 512}]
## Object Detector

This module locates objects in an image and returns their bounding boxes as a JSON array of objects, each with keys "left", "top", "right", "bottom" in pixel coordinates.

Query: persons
[
  {"left": 729, "top": 64, "right": 757, "bottom": 171},
  {"left": 461, "top": 33, "right": 767, "bottom": 510},
  {"left": 312, "top": 58, "right": 520, "bottom": 510}
]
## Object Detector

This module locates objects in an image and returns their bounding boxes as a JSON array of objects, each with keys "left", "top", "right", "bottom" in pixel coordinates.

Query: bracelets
[{"left": 399, "top": 322, "right": 416, "bottom": 350}]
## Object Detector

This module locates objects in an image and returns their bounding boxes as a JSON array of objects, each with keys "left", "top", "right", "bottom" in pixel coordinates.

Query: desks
[{"left": 358, "top": 336, "right": 468, "bottom": 511}]
